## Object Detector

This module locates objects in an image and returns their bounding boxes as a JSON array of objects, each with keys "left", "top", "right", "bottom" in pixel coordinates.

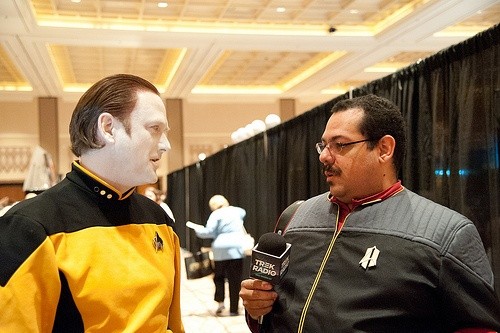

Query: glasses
[{"left": 315, "top": 138, "right": 368, "bottom": 155}]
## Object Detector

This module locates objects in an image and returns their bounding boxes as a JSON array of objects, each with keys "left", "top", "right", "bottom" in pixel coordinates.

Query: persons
[
  {"left": 194, "top": 194, "right": 255, "bottom": 316},
  {"left": 0, "top": 75, "right": 185, "bottom": 333},
  {"left": 237, "top": 94, "right": 500, "bottom": 333}
]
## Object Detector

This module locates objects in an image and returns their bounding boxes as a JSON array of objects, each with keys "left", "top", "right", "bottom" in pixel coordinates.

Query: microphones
[{"left": 248, "top": 232, "right": 292, "bottom": 333}]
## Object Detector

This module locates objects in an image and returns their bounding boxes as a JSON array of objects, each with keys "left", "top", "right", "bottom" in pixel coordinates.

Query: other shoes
[
  {"left": 215, "top": 305, "right": 226, "bottom": 314},
  {"left": 229, "top": 313, "right": 239, "bottom": 316}
]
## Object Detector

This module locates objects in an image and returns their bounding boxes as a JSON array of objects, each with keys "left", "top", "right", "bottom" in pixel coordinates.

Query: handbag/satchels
[{"left": 184, "top": 247, "right": 213, "bottom": 281}]
